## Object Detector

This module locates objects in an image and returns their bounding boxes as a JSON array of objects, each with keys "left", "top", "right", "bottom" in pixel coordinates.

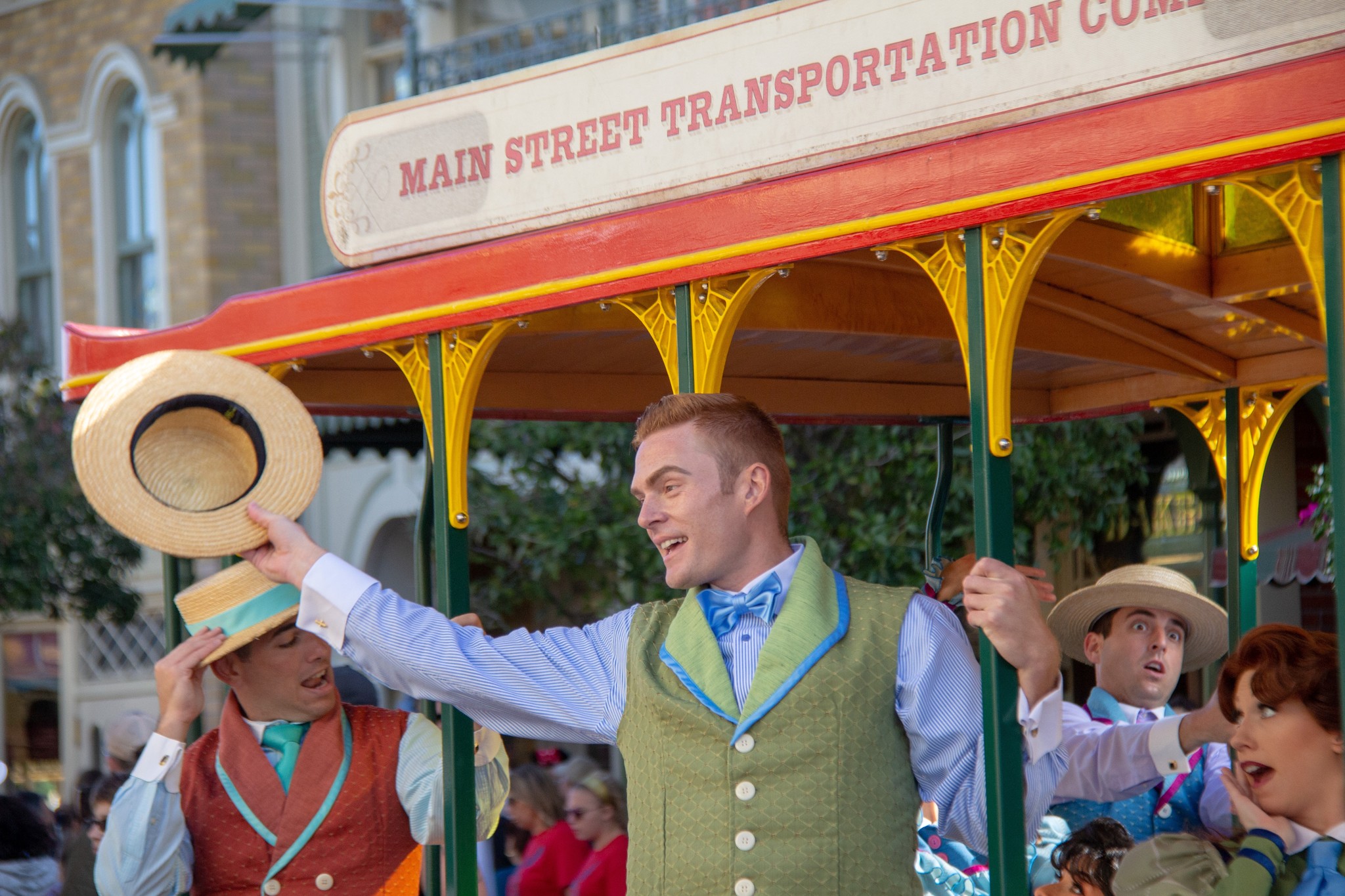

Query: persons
[
  {"left": 497, "top": 751, "right": 626, "bottom": 896},
  {"left": 243, "top": 388, "right": 1064, "bottom": 896},
  {"left": 0, "top": 716, "right": 148, "bottom": 896},
  {"left": 1038, "top": 566, "right": 1319, "bottom": 856},
  {"left": 915, "top": 798, "right": 1138, "bottom": 896},
  {"left": 93, "top": 555, "right": 509, "bottom": 896},
  {"left": 1209, "top": 621, "right": 1344, "bottom": 896}
]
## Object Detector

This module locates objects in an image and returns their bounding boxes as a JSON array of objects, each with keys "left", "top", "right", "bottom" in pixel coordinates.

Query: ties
[
  {"left": 1290, "top": 839, "right": 1345, "bottom": 896},
  {"left": 262, "top": 724, "right": 310, "bottom": 797}
]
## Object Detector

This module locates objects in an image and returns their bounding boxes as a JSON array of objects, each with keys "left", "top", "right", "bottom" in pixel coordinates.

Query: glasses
[
  {"left": 82, "top": 818, "right": 106, "bottom": 833},
  {"left": 506, "top": 797, "right": 604, "bottom": 820}
]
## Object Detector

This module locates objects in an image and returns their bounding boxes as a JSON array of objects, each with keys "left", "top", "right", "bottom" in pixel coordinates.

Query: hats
[
  {"left": 1046, "top": 563, "right": 1231, "bottom": 676},
  {"left": 71, "top": 349, "right": 325, "bottom": 558},
  {"left": 172, "top": 559, "right": 302, "bottom": 672}
]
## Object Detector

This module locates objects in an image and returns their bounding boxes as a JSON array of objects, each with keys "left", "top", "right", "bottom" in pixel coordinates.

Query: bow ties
[{"left": 696, "top": 570, "right": 782, "bottom": 641}]
